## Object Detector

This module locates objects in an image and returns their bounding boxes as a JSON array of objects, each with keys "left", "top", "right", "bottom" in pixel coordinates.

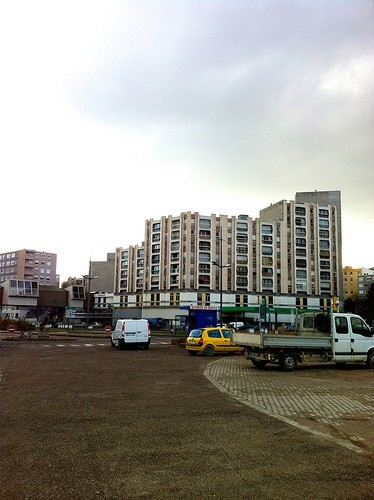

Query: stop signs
[
  {"left": 105, "top": 326, "right": 111, "bottom": 332},
  {"left": 8, "top": 325, "right": 16, "bottom": 333}
]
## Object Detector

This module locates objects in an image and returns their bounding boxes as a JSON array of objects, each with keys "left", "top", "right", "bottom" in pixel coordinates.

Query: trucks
[{"left": 232, "top": 312, "right": 374, "bottom": 373}]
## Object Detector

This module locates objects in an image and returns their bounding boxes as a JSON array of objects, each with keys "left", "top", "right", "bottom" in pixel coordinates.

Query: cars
[
  {"left": 185, "top": 327, "right": 245, "bottom": 357},
  {"left": 53, "top": 322, "right": 67, "bottom": 328},
  {"left": 73, "top": 322, "right": 103, "bottom": 327}
]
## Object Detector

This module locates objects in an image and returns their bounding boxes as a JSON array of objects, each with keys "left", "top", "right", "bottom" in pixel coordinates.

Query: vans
[{"left": 110, "top": 318, "right": 152, "bottom": 349}]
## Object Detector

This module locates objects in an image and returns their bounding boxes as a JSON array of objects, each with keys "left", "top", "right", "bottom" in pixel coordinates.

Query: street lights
[
  {"left": 83, "top": 275, "right": 99, "bottom": 326},
  {"left": 211, "top": 261, "right": 231, "bottom": 328}
]
